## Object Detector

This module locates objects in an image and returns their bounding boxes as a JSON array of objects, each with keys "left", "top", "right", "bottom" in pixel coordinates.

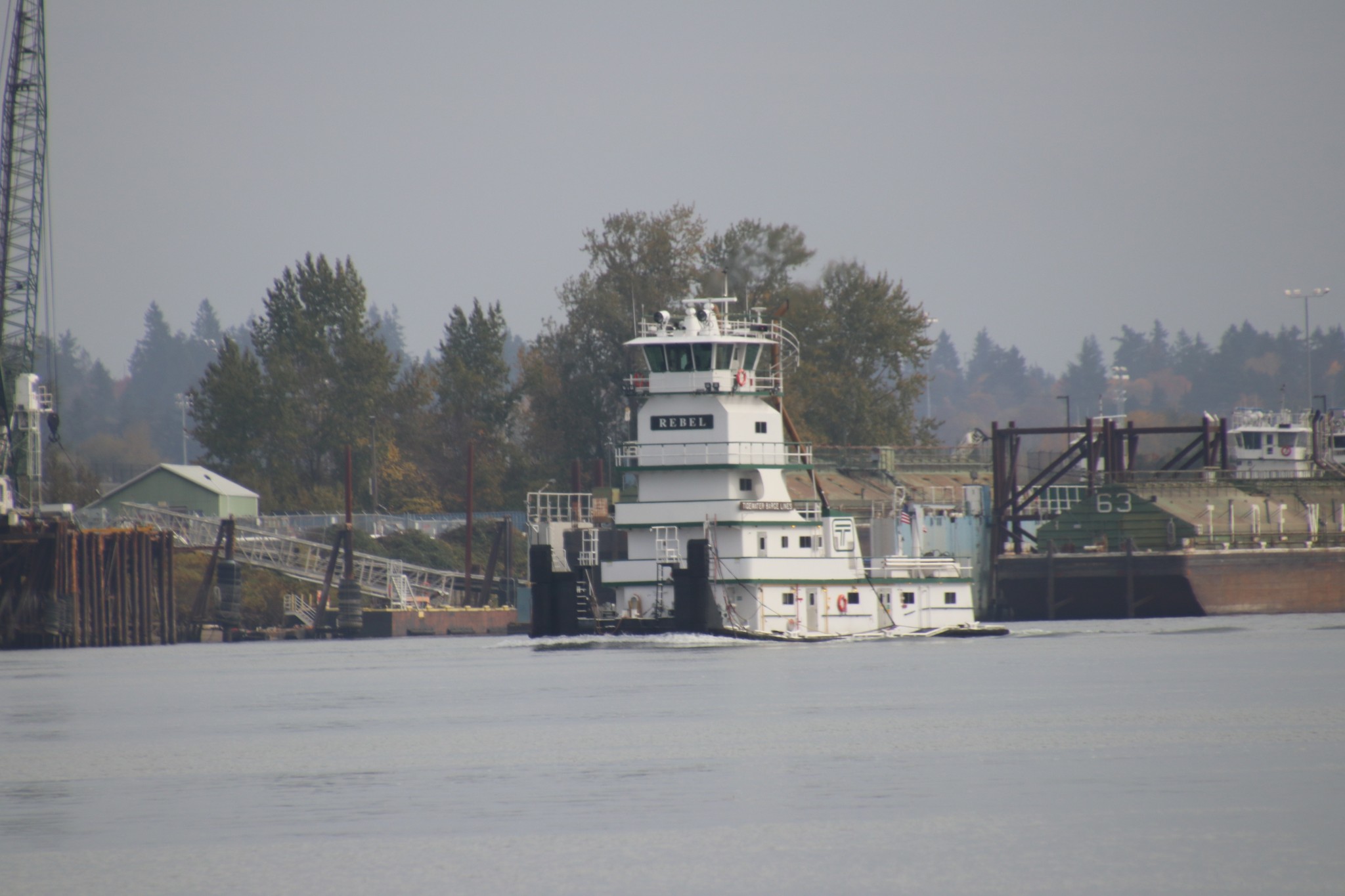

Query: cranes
[{"left": 0, "top": 0, "right": 62, "bottom": 527}]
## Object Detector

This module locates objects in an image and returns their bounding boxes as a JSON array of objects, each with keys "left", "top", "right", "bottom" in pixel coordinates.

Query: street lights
[
  {"left": 1283, "top": 286, "right": 1330, "bottom": 415},
  {"left": 172, "top": 392, "right": 194, "bottom": 466},
  {"left": 1054, "top": 394, "right": 1070, "bottom": 450},
  {"left": 920, "top": 310, "right": 939, "bottom": 436},
  {"left": 1113, "top": 364, "right": 1129, "bottom": 425}
]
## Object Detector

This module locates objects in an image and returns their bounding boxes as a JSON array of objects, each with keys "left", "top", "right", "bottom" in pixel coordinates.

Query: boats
[{"left": 525, "top": 266, "right": 1009, "bottom": 649}]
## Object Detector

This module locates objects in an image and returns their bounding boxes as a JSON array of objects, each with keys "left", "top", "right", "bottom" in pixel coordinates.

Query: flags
[{"left": 901, "top": 502, "right": 927, "bottom": 532}]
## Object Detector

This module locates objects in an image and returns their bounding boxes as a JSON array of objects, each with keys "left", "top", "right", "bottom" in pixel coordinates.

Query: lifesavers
[
  {"left": 737, "top": 368, "right": 747, "bottom": 385},
  {"left": 634, "top": 373, "right": 644, "bottom": 386},
  {"left": 838, "top": 595, "right": 847, "bottom": 612},
  {"left": 1281, "top": 446, "right": 1291, "bottom": 456},
  {"left": 627, "top": 594, "right": 641, "bottom": 611}
]
